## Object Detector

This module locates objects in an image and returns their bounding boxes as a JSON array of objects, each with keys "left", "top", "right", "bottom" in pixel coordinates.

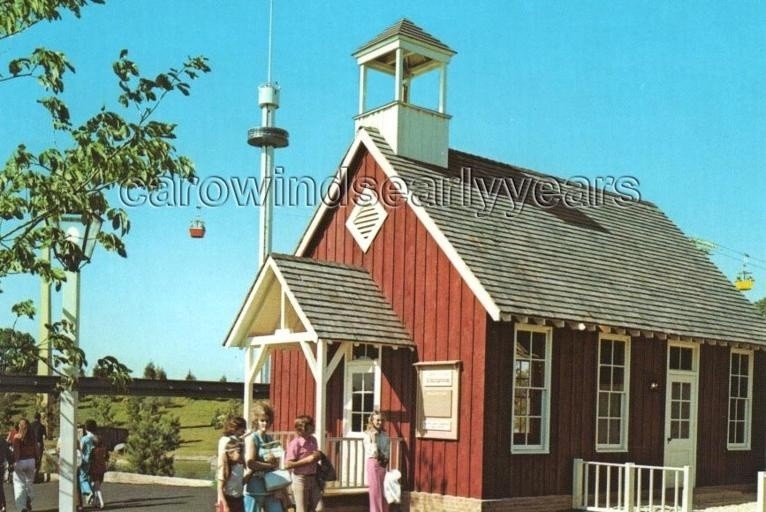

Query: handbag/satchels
[
  {"left": 383, "top": 469, "right": 403, "bottom": 504},
  {"left": 317, "top": 458, "right": 336, "bottom": 482},
  {"left": 264, "top": 470, "right": 291, "bottom": 491}
]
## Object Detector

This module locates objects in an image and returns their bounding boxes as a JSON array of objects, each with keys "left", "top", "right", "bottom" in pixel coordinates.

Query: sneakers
[{"left": 86, "top": 492, "right": 94, "bottom": 504}]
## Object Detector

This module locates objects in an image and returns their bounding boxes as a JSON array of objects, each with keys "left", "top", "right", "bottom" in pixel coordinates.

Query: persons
[
  {"left": 0, "top": 410, "right": 48, "bottom": 512},
  {"left": 363, "top": 409, "right": 391, "bottom": 512},
  {"left": 56, "top": 419, "right": 107, "bottom": 512},
  {"left": 215, "top": 400, "right": 327, "bottom": 512}
]
